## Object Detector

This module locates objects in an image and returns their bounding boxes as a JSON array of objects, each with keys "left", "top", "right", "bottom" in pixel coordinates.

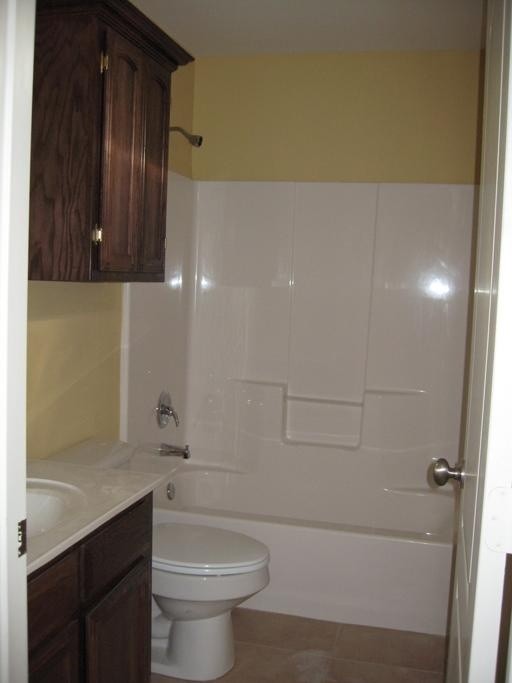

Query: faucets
[{"left": 160, "top": 441, "right": 191, "bottom": 460}]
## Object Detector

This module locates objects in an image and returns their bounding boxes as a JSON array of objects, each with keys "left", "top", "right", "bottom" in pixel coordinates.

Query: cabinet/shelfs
[
  {"left": 27, "top": 491, "right": 153, "bottom": 683},
  {"left": 29, "top": 0, "right": 196, "bottom": 283}
]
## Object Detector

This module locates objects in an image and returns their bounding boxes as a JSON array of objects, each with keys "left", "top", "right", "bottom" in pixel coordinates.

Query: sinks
[
  {"left": 150, "top": 464, "right": 456, "bottom": 637},
  {"left": 27, "top": 475, "right": 86, "bottom": 543}
]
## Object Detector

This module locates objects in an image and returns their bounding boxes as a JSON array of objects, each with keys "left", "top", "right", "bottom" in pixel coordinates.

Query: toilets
[{"left": 43, "top": 434, "right": 269, "bottom": 682}]
